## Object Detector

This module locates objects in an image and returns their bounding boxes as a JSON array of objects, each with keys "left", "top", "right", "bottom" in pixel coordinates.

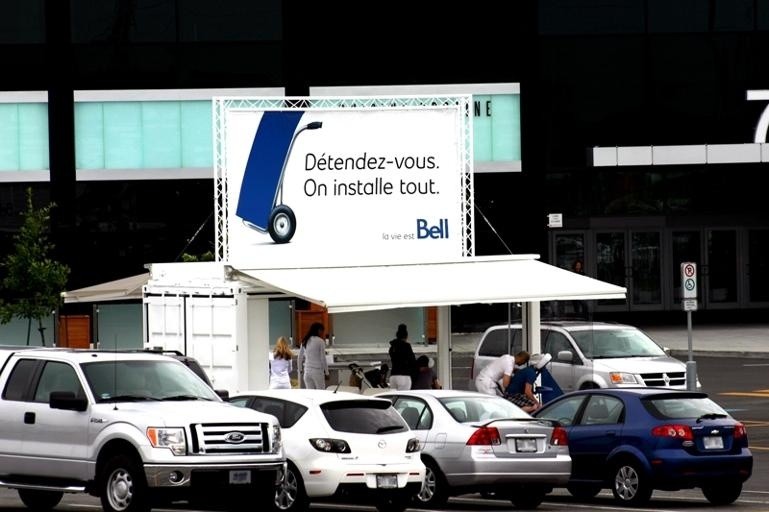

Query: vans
[{"left": 471, "top": 320, "right": 700, "bottom": 395}]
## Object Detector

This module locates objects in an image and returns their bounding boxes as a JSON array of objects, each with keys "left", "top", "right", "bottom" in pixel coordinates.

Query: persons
[
  {"left": 504, "top": 353, "right": 551, "bottom": 412},
  {"left": 573, "top": 252, "right": 585, "bottom": 316},
  {"left": 356, "top": 364, "right": 388, "bottom": 388},
  {"left": 302, "top": 322, "right": 330, "bottom": 389},
  {"left": 416, "top": 355, "right": 441, "bottom": 389},
  {"left": 475, "top": 351, "right": 530, "bottom": 396},
  {"left": 388, "top": 323, "right": 416, "bottom": 390},
  {"left": 269, "top": 335, "right": 294, "bottom": 389}
]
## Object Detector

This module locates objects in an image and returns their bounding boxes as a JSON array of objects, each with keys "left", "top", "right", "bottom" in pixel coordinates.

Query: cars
[
  {"left": 375, "top": 389, "right": 575, "bottom": 508},
  {"left": 531, "top": 387, "right": 753, "bottom": 508},
  {"left": 225, "top": 388, "right": 428, "bottom": 512}
]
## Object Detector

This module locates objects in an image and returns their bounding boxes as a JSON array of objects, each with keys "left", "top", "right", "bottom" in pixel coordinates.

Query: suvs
[
  {"left": 0, "top": 347, "right": 289, "bottom": 510},
  {"left": 94, "top": 349, "right": 229, "bottom": 401}
]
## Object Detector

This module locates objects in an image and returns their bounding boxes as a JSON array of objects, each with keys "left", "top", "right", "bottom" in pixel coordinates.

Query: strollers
[{"left": 349, "top": 362, "right": 390, "bottom": 388}]
